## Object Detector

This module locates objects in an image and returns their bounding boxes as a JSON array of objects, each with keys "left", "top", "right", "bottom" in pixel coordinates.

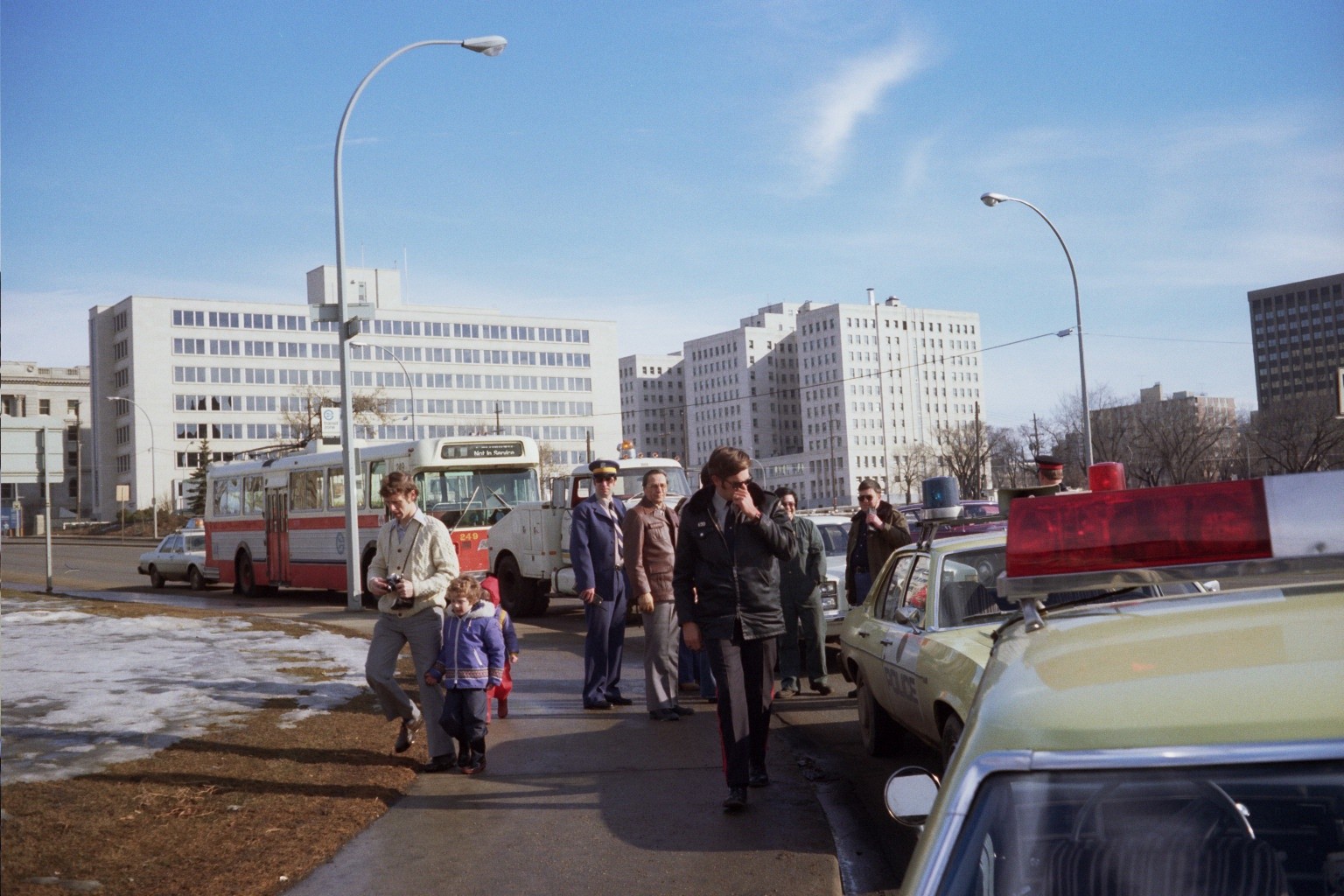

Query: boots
[
  {"left": 462, "top": 736, "right": 487, "bottom": 773},
  {"left": 457, "top": 739, "right": 471, "bottom": 768}
]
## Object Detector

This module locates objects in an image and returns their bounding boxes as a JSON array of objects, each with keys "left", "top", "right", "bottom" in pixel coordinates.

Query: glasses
[
  {"left": 782, "top": 502, "right": 796, "bottom": 507},
  {"left": 647, "top": 484, "right": 666, "bottom": 489},
  {"left": 857, "top": 495, "right": 877, "bottom": 502},
  {"left": 593, "top": 477, "right": 615, "bottom": 483},
  {"left": 716, "top": 473, "right": 753, "bottom": 491}
]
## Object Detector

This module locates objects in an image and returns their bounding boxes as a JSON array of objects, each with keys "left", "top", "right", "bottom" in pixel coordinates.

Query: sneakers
[
  {"left": 425, "top": 753, "right": 457, "bottom": 773},
  {"left": 726, "top": 788, "right": 747, "bottom": 807},
  {"left": 668, "top": 705, "right": 694, "bottom": 715},
  {"left": 650, "top": 709, "right": 679, "bottom": 720},
  {"left": 395, "top": 713, "right": 424, "bottom": 753},
  {"left": 749, "top": 771, "right": 769, "bottom": 785}
]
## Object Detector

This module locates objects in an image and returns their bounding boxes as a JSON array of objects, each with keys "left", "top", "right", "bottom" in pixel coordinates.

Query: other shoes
[
  {"left": 498, "top": 697, "right": 508, "bottom": 719},
  {"left": 485, "top": 713, "right": 491, "bottom": 724},
  {"left": 848, "top": 689, "right": 857, "bottom": 697},
  {"left": 810, "top": 683, "right": 834, "bottom": 694},
  {"left": 708, "top": 698, "right": 718, "bottom": 703},
  {"left": 775, "top": 688, "right": 800, "bottom": 698},
  {"left": 683, "top": 684, "right": 700, "bottom": 691}
]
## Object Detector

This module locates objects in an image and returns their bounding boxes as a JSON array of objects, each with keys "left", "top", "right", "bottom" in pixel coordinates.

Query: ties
[
  {"left": 724, "top": 501, "right": 736, "bottom": 560},
  {"left": 609, "top": 502, "right": 624, "bottom": 558}
]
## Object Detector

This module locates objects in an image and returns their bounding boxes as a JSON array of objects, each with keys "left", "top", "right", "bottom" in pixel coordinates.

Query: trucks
[{"left": 486, "top": 441, "right": 695, "bottom": 619}]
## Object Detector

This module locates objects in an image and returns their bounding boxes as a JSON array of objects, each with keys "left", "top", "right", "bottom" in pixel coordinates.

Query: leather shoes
[
  {"left": 584, "top": 701, "right": 615, "bottom": 709},
  {"left": 606, "top": 698, "right": 632, "bottom": 705}
]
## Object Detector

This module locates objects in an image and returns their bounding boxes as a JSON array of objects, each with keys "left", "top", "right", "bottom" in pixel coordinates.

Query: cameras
[{"left": 381, "top": 573, "right": 401, "bottom": 593}]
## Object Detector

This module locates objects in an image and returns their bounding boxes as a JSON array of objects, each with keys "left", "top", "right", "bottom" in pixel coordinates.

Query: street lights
[
  {"left": 750, "top": 458, "right": 766, "bottom": 490},
  {"left": 350, "top": 342, "right": 417, "bottom": 441},
  {"left": 105, "top": 396, "right": 158, "bottom": 539},
  {"left": 980, "top": 193, "right": 1093, "bottom": 470},
  {"left": 334, "top": 35, "right": 508, "bottom": 609}
]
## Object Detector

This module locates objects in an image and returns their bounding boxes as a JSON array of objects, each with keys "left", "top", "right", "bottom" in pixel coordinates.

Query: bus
[{"left": 203, "top": 434, "right": 544, "bottom": 607}]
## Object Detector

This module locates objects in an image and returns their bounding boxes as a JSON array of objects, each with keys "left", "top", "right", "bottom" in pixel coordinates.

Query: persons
[
  {"left": 672, "top": 445, "right": 798, "bottom": 812},
  {"left": 664, "top": 484, "right": 835, "bottom": 710},
  {"left": 623, "top": 468, "right": 694, "bottom": 720},
  {"left": 423, "top": 574, "right": 506, "bottom": 774},
  {"left": 569, "top": 459, "right": 633, "bottom": 710},
  {"left": 1034, "top": 455, "right": 1069, "bottom": 494},
  {"left": 844, "top": 480, "right": 913, "bottom": 700},
  {"left": 365, "top": 471, "right": 462, "bottom": 772},
  {"left": 480, "top": 576, "right": 519, "bottom": 724},
  {"left": 968, "top": 507, "right": 993, "bottom": 527}
]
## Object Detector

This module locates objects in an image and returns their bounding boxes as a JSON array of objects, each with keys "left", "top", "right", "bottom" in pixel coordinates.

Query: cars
[
  {"left": 895, "top": 457, "right": 1343, "bottom": 896},
  {"left": 138, "top": 518, "right": 220, "bottom": 591},
  {"left": 895, "top": 500, "right": 1008, "bottom": 544},
  {"left": 840, "top": 476, "right": 1221, "bottom": 770},
  {"left": 799, "top": 514, "right": 854, "bottom": 642}
]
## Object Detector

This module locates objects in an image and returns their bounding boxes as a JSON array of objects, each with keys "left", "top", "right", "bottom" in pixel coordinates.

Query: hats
[
  {"left": 588, "top": 460, "right": 620, "bottom": 478},
  {"left": 1035, "top": 456, "right": 1065, "bottom": 473}
]
